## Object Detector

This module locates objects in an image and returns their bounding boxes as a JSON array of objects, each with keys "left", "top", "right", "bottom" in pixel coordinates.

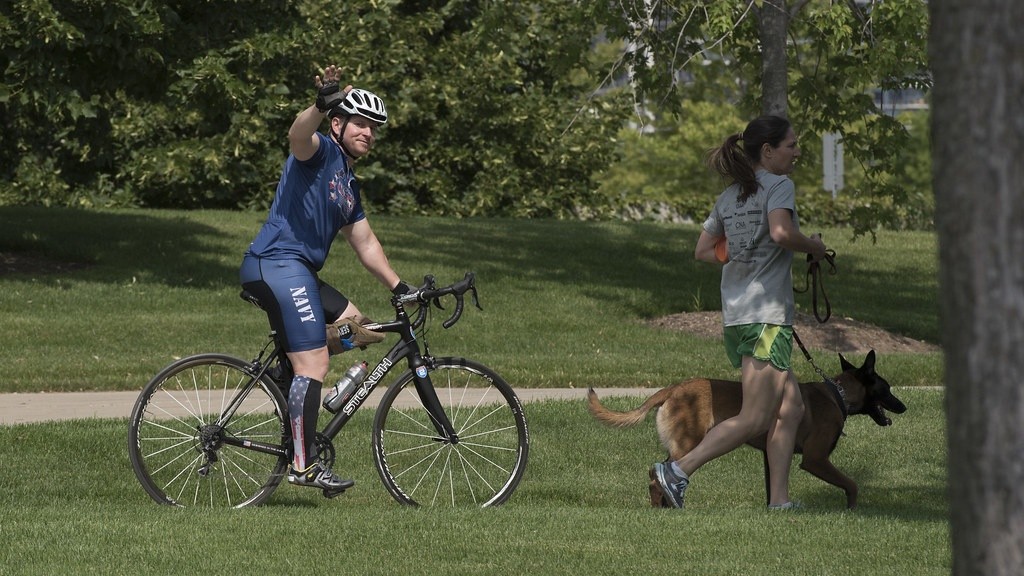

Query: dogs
[{"left": 585, "top": 349, "right": 908, "bottom": 508}]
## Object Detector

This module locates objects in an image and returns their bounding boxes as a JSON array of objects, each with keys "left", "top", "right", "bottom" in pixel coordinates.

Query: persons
[
  {"left": 648, "top": 115, "right": 825, "bottom": 511},
  {"left": 237, "top": 64, "right": 417, "bottom": 489}
]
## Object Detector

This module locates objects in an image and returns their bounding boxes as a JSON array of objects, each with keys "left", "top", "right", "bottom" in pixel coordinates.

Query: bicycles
[{"left": 126, "top": 270, "right": 530, "bottom": 513}]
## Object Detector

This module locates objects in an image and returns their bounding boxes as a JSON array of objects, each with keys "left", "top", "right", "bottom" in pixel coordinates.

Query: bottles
[{"left": 322, "top": 360, "right": 368, "bottom": 414}]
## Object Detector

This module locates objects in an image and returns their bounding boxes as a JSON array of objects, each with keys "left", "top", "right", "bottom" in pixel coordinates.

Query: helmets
[{"left": 327, "top": 89, "right": 387, "bottom": 127}]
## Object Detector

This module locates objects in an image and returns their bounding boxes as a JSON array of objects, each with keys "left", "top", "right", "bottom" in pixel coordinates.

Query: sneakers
[
  {"left": 257, "top": 366, "right": 283, "bottom": 416},
  {"left": 768, "top": 502, "right": 799, "bottom": 510},
  {"left": 288, "top": 461, "right": 354, "bottom": 489},
  {"left": 649, "top": 462, "right": 689, "bottom": 509}
]
